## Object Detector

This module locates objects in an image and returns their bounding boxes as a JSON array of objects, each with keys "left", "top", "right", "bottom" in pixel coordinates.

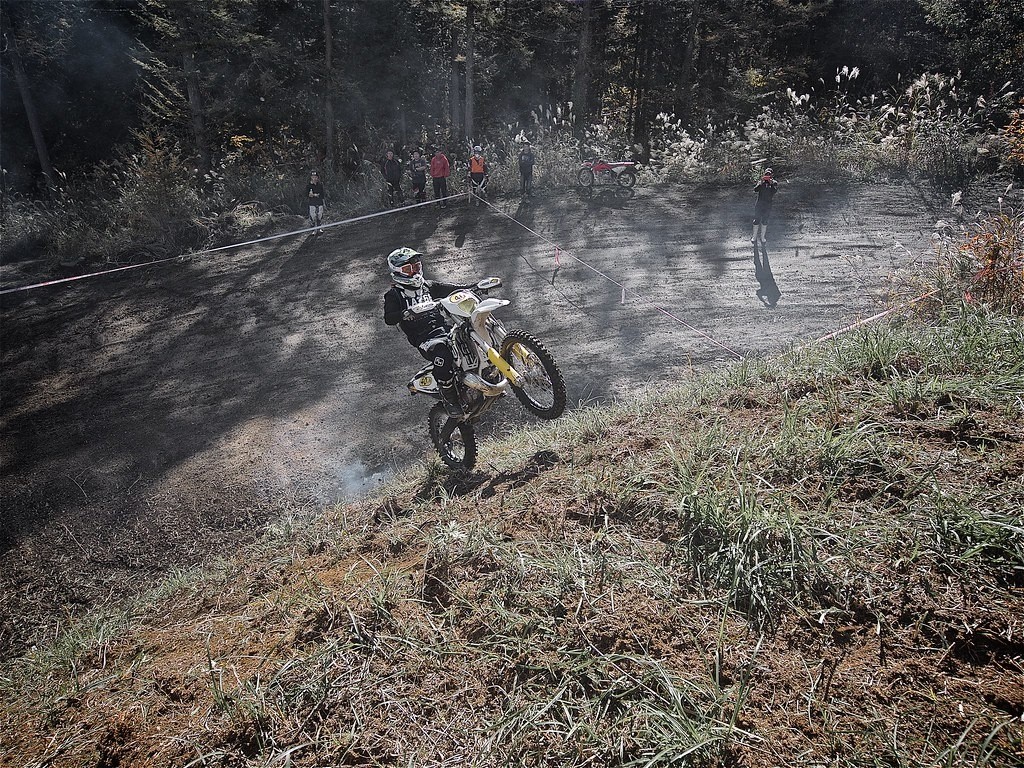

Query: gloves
[
  {"left": 401, "top": 309, "right": 417, "bottom": 323},
  {"left": 467, "top": 173, "right": 471, "bottom": 180},
  {"left": 484, "top": 172, "right": 488, "bottom": 178}
]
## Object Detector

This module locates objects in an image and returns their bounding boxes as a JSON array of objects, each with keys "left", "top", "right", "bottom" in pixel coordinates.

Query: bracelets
[
  {"left": 315, "top": 193, "right": 317, "bottom": 197},
  {"left": 414, "top": 169, "right": 418, "bottom": 173}
]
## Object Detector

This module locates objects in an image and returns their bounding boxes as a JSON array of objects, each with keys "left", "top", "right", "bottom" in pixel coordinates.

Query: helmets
[
  {"left": 386, "top": 247, "right": 424, "bottom": 288},
  {"left": 473, "top": 146, "right": 482, "bottom": 157}
]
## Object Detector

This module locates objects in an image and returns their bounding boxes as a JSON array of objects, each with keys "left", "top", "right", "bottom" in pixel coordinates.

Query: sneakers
[{"left": 439, "top": 388, "right": 463, "bottom": 418}]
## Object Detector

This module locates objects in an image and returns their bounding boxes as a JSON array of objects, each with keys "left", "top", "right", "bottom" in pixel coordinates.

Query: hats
[
  {"left": 310, "top": 171, "right": 318, "bottom": 176},
  {"left": 765, "top": 168, "right": 773, "bottom": 175}
]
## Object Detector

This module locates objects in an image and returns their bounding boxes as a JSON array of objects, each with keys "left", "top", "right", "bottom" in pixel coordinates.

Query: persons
[
  {"left": 409, "top": 151, "right": 428, "bottom": 208},
  {"left": 303, "top": 171, "right": 326, "bottom": 236},
  {"left": 467, "top": 146, "right": 488, "bottom": 207},
  {"left": 518, "top": 143, "right": 534, "bottom": 203},
  {"left": 751, "top": 168, "right": 778, "bottom": 243},
  {"left": 430, "top": 149, "right": 450, "bottom": 208},
  {"left": 381, "top": 149, "right": 407, "bottom": 208},
  {"left": 384, "top": 248, "right": 507, "bottom": 418}
]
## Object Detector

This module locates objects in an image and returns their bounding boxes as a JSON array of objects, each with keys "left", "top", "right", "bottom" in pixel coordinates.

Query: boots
[
  {"left": 751, "top": 224, "right": 760, "bottom": 242},
  {"left": 760, "top": 223, "right": 767, "bottom": 243}
]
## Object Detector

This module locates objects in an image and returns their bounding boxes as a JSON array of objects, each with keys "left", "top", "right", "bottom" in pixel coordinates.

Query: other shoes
[
  {"left": 312, "top": 230, "right": 317, "bottom": 235},
  {"left": 485, "top": 199, "right": 490, "bottom": 208},
  {"left": 417, "top": 202, "right": 422, "bottom": 207},
  {"left": 519, "top": 199, "right": 524, "bottom": 204},
  {"left": 475, "top": 199, "right": 480, "bottom": 206},
  {"left": 319, "top": 229, "right": 323, "bottom": 232},
  {"left": 524, "top": 197, "right": 530, "bottom": 204},
  {"left": 401, "top": 209, "right": 408, "bottom": 212},
  {"left": 441, "top": 203, "right": 446, "bottom": 209},
  {"left": 434, "top": 204, "right": 440, "bottom": 208}
]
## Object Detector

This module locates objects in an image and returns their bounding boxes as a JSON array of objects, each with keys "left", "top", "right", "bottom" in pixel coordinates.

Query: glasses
[{"left": 387, "top": 257, "right": 423, "bottom": 276}]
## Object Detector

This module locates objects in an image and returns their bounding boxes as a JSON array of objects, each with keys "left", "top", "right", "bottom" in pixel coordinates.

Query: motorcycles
[
  {"left": 579, "top": 154, "right": 637, "bottom": 189},
  {"left": 409, "top": 286, "right": 568, "bottom": 473}
]
